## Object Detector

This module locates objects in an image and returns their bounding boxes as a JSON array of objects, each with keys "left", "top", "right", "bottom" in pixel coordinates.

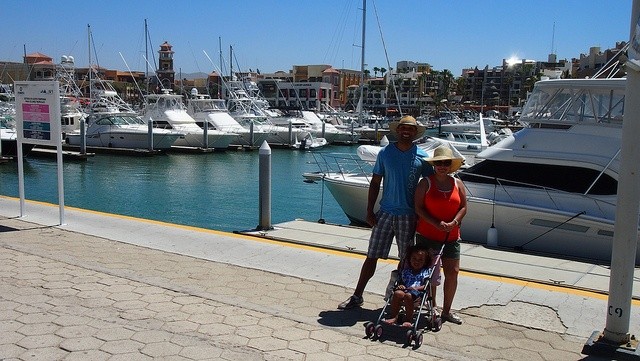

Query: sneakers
[
  {"left": 432, "top": 308, "right": 440, "bottom": 317},
  {"left": 338, "top": 296, "right": 364, "bottom": 309},
  {"left": 441, "top": 312, "right": 462, "bottom": 323}
]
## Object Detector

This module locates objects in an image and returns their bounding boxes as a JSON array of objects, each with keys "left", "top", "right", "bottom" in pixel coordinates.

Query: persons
[
  {"left": 385, "top": 245, "right": 432, "bottom": 329},
  {"left": 338, "top": 116, "right": 431, "bottom": 310},
  {"left": 414, "top": 147, "right": 468, "bottom": 324}
]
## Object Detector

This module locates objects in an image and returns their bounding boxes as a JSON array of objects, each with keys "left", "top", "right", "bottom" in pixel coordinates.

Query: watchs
[{"left": 453, "top": 219, "right": 458, "bottom": 227}]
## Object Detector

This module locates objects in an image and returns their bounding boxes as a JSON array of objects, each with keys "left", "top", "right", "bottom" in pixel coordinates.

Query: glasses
[{"left": 433, "top": 161, "right": 452, "bottom": 166}]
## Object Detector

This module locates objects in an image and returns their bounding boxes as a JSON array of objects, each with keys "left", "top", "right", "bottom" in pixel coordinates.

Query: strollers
[{"left": 364, "top": 229, "right": 449, "bottom": 348}]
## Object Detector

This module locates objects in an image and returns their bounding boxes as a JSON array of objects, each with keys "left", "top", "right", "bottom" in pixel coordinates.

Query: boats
[
  {"left": 438, "top": 117, "right": 500, "bottom": 135},
  {"left": 202, "top": 47, "right": 314, "bottom": 149},
  {"left": 136, "top": 18, "right": 241, "bottom": 149},
  {"left": 186, "top": 86, "right": 269, "bottom": 151},
  {"left": 271, "top": 74, "right": 361, "bottom": 145},
  {"left": 305, "top": 40, "right": 640, "bottom": 265},
  {"left": 66, "top": 21, "right": 187, "bottom": 149}
]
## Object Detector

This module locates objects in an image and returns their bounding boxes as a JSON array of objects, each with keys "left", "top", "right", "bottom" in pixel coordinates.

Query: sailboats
[{"left": 0, "top": 81, "right": 36, "bottom": 158}]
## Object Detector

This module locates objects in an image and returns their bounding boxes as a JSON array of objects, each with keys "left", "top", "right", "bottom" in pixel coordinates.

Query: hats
[
  {"left": 423, "top": 146, "right": 462, "bottom": 172},
  {"left": 390, "top": 116, "right": 425, "bottom": 140}
]
